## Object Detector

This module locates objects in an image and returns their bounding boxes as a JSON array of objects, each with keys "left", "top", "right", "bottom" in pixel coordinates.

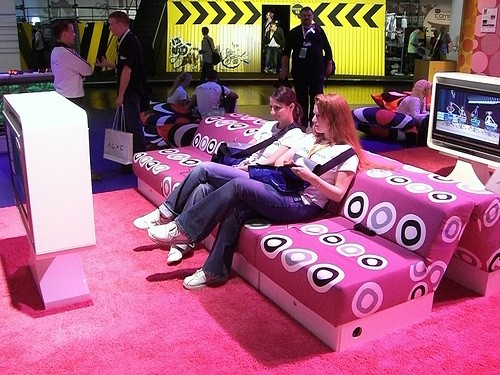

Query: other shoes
[
  {"left": 263, "top": 69, "right": 268, "bottom": 74},
  {"left": 91, "top": 171, "right": 101, "bottom": 181},
  {"left": 269, "top": 69, "right": 276, "bottom": 73}
]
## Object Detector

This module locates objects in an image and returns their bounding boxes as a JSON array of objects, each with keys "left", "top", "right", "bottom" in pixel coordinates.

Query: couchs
[
  {"left": 350, "top": 105, "right": 422, "bottom": 143},
  {"left": 139, "top": 102, "right": 205, "bottom": 147}
]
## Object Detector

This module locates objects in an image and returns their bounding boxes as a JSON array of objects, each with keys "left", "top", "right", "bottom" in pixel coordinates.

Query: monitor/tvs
[{"left": 428, "top": 71, "right": 500, "bottom": 169}]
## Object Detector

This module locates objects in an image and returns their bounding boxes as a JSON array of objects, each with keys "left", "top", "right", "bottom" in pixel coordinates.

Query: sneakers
[
  {"left": 167, "top": 241, "right": 195, "bottom": 266},
  {"left": 147, "top": 221, "right": 191, "bottom": 244},
  {"left": 134, "top": 208, "right": 173, "bottom": 230},
  {"left": 183, "top": 268, "right": 221, "bottom": 290}
]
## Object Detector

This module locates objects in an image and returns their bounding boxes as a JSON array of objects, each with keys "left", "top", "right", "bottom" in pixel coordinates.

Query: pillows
[{"left": 370, "top": 91, "right": 412, "bottom": 110}]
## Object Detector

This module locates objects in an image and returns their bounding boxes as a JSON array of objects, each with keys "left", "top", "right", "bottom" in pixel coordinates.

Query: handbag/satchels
[
  {"left": 34, "top": 48, "right": 50, "bottom": 61},
  {"left": 323, "top": 55, "right": 336, "bottom": 77},
  {"left": 211, "top": 141, "right": 248, "bottom": 166},
  {"left": 248, "top": 165, "right": 305, "bottom": 194},
  {"left": 103, "top": 104, "right": 134, "bottom": 165},
  {"left": 212, "top": 51, "right": 221, "bottom": 65}
]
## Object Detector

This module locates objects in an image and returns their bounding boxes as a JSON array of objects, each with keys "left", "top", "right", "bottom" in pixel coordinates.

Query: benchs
[
  {"left": 132, "top": 117, "right": 475, "bottom": 352},
  {"left": 227, "top": 113, "right": 500, "bottom": 297}
]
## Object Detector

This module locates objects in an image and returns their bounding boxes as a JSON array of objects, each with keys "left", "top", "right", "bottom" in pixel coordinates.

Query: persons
[
  {"left": 262, "top": 12, "right": 284, "bottom": 74},
  {"left": 199, "top": 27, "right": 214, "bottom": 83},
  {"left": 133, "top": 86, "right": 308, "bottom": 267},
  {"left": 398, "top": 79, "right": 431, "bottom": 125},
  {"left": 166, "top": 70, "right": 238, "bottom": 119},
  {"left": 33, "top": 22, "right": 45, "bottom": 69},
  {"left": 280, "top": 7, "right": 332, "bottom": 128},
  {"left": 50, "top": 20, "right": 102, "bottom": 181},
  {"left": 147, "top": 93, "right": 396, "bottom": 290},
  {"left": 407, "top": 27, "right": 423, "bottom": 76},
  {"left": 430, "top": 26, "right": 451, "bottom": 60},
  {"left": 93, "top": 11, "right": 143, "bottom": 173}
]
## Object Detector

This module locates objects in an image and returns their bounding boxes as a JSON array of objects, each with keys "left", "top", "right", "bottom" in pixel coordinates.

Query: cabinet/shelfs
[
  {"left": 413, "top": 59, "right": 456, "bottom": 83},
  {"left": 386, "top": 13, "right": 407, "bottom": 76}
]
